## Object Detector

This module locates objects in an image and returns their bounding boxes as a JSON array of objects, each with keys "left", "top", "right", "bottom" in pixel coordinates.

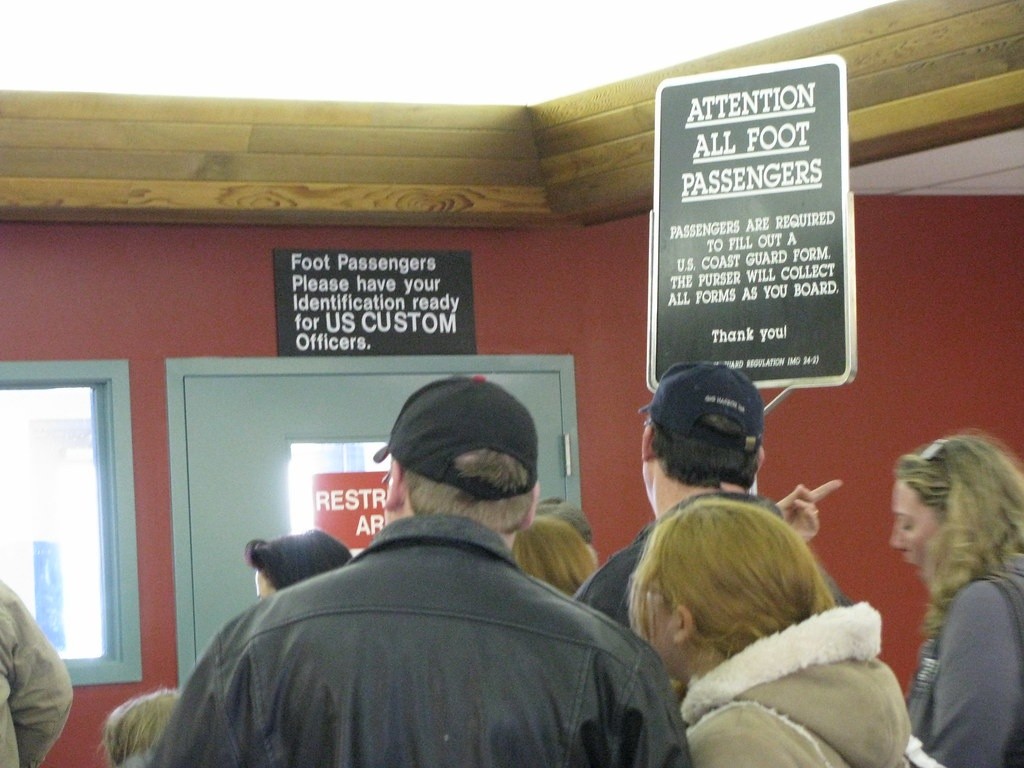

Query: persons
[
  {"left": 103, "top": 686, "right": 188, "bottom": 768},
  {"left": 245, "top": 529, "right": 352, "bottom": 601},
  {"left": 510, "top": 354, "right": 1024, "bottom": 768},
  {"left": 151, "top": 374, "right": 691, "bottom": 768},
  {"left": 0, "top": 580, "right": 73, "bottom": 768}
]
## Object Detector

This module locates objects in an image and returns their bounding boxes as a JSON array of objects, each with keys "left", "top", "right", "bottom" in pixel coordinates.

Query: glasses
[{"left": 910, "top": 438, "right": 946, "bottom": 462}]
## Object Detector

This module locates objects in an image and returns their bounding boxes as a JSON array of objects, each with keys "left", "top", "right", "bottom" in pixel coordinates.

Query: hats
[
  {"left": 636, "top": 360, "right": 764, "bottom": 453},
  {"left": 372, "top": 376, "right": 540, "bottom": 500}
]
